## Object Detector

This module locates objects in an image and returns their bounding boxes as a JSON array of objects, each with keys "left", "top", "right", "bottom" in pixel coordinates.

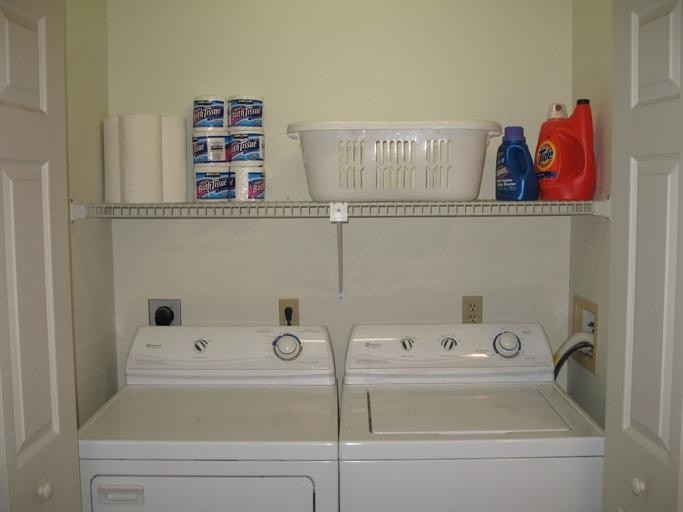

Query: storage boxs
[{"left": 286, "top": 119, "right": 502, "bottom": 204}]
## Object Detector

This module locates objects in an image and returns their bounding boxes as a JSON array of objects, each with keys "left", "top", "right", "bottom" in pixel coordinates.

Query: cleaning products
[
  {"left": 494, "top": 125, "right": 537, "bottom": 199},
  {"left": 532, "top": 98, "right": 597, "bottom": 199}
]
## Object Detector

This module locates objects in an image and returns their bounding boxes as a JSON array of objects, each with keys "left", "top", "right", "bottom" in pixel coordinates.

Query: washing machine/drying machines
[
  {"left": 77, "top": 322, "right": 338, "bottom": 511},
  {"left": 337, "top": 321, "right": 606, "bottom": 511}
]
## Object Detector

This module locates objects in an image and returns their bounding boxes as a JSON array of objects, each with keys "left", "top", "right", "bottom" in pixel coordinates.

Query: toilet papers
[
  {"left": 99, "top": 115, "right": 120, "bottom": 205},
  {"left": 162, "top": 115, "right": 189, "bottom": 203},
  {"left": 119, "top": 110, "right": 162, "bottom": 203},
  {"left": 191, "top": 95, "right": 265, "bottom": 202}
]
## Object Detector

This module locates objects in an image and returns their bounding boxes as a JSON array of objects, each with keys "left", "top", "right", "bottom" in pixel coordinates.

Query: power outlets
[{"left": 462, "top": 292, "right": 484, "bottom": 321}]
